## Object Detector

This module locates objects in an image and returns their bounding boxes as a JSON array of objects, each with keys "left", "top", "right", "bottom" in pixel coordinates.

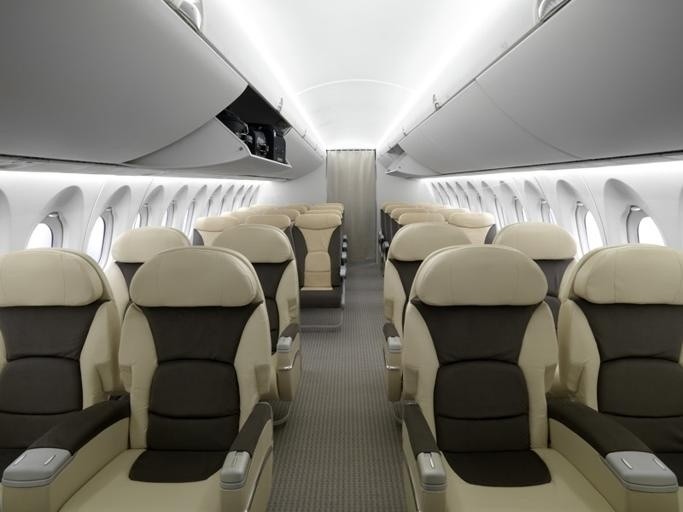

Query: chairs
[
  {"left": 0, "top": 249, "right": 117, "bottom": 511},
  {"left": 191, "top": 203, "right": 308, "bottom": 247},
  {"left": 102, "top": 226, "right": 190, "bottom": 319},
  {"left": 291, "top": 212, "right": 348, "bottom": 332},
  {"left": 204, "top": 223, "right": 301, "bottom": 428},
  {"left": 377, "top": 200, "right": 495, "bottom": 243},
  {"left": 1, "top": 245, "right": 278, "bottom": 511},
  {"left": 398, "top": 247, "right": 681, "bottom": 512},
  {"left": 558, "top": 244, "right": 683, "bottom": 508},
  {"left": 493, "top": 222, "right": 579, "bottom": 320},
  {"left": 382, "top": 223, "right": 474, "bottom": 405}
]
